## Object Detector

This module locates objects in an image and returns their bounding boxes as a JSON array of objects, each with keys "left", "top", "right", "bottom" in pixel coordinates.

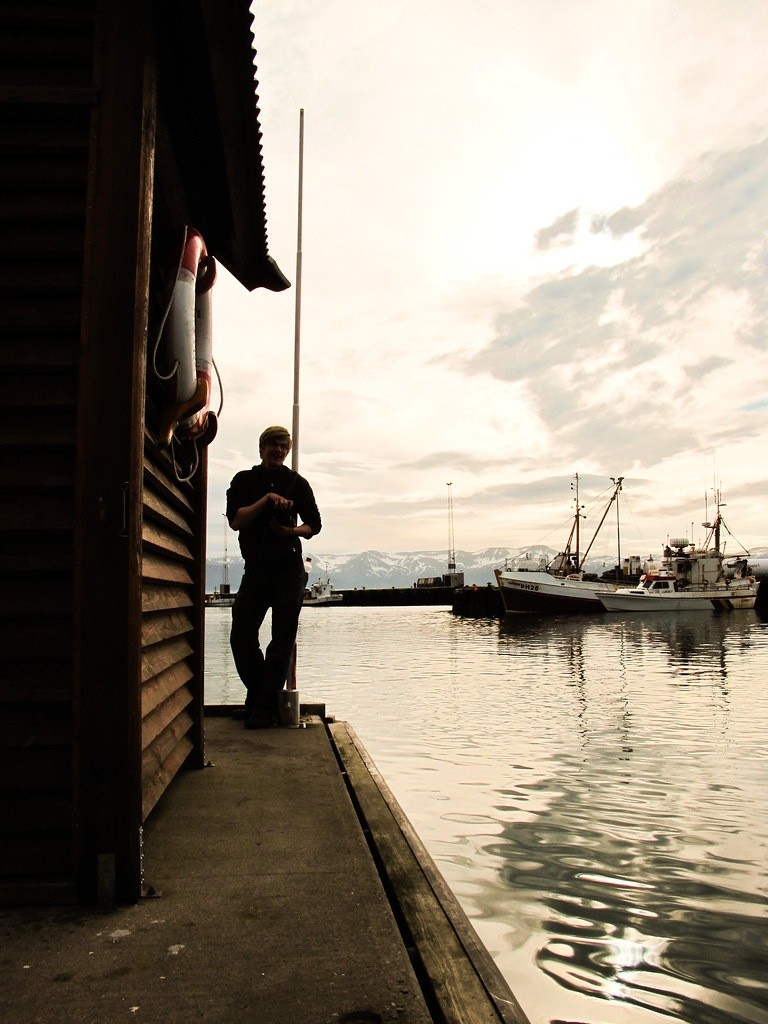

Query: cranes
[
  {"left": 445, "top": 482, "right": 456, "bottom": 574},
  {"left": 222, "top": 513, "right": 230, "bottom": 583}
]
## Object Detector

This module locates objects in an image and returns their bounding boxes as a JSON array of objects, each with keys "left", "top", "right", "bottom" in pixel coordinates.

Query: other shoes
[{"left": 231, "top": 697, "right": 272, "bottom": 730}]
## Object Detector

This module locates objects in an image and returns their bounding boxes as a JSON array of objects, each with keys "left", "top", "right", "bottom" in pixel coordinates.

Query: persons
[{"left": 226, "top": 426, "right": 322, "bottom": 731}]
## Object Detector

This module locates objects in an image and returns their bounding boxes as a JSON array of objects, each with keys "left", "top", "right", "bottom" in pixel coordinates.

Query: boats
[
  {"left": 494, "top": 472, "right": 768, "bottom": 619},
  {"left": 204, "top": 586, "right": 235, "bottom": 606},
  {"left": 303, "top": 578, "right": 343, "bottom": 605}
]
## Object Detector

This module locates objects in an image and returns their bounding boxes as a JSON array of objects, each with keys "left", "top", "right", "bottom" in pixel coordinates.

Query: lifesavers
[{"left": 170, "top": 226, "right": 214, "bottom": 436}]
